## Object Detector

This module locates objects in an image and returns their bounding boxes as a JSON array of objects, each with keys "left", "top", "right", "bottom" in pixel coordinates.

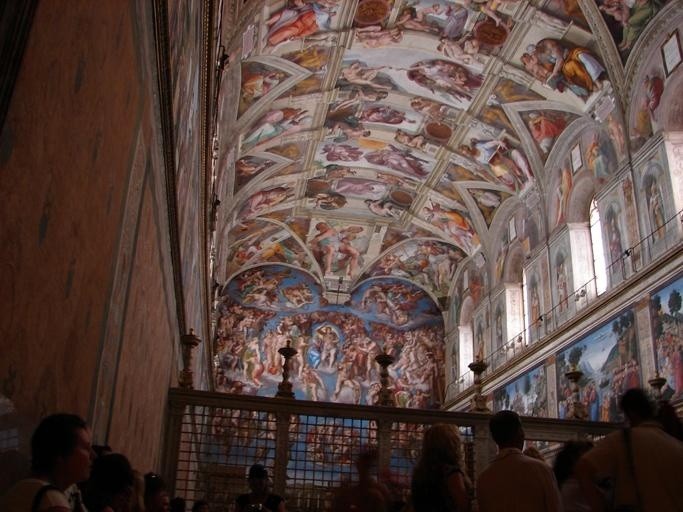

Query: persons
[
  {"left": 332, "top": 448, "right": 393, "bottom": 508},
  {"left": 553, "top": 440, "right": 616, "bottom": 508},
  {"left": 475, "top": 410, "right": 564, "bottom": 508},
  {"left": 234, "top": 466, "right": 287, "bottom": 508},
  {"left": 578, "top": 387, "right": 680, "bottom": 508},
  {"left": 192, "top": 500, "right": 209, "bottom": 508},
  {"left": 3, "top": 413, "right": 186, "bottom": 509},
  {"left": 409, "top": 423, "right": 474, "bottom": 508}
]
